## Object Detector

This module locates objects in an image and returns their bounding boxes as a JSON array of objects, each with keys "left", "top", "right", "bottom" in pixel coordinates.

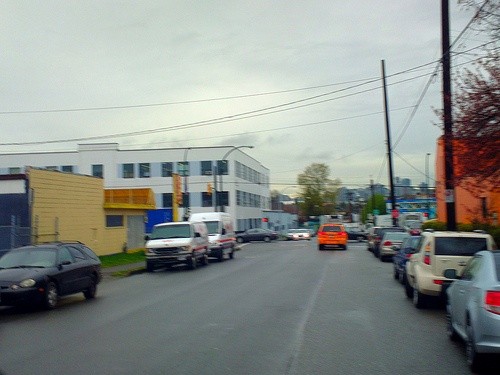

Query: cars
[
  {"left": 405, "top": 228, "right": 494, "bottom": 307},
  {"left": 294, "top": 229, "right": 311, "bottom": 240},
  {"left": 371, "top": 227, "right": 406, "bottom": 256},
  {"left": 346, "top": 226, "right": 371, "bottom": 242},
  {"left": 379, "top": 232, "right": 412, "bottom": 261},
  {"left": 393, "top": 235, "right": 423, "bottom": 284},
  {"left": 443, "top": 250, "right": 500, "bottom": 371},
  {"left": 0, "top": 240, "right": 102, "bottom": 309},
  {"left": 235, "top": 227, "right": 279, "bottom": 242},
  {"left": 308, "top": 229, "right": 315, "bottom": 236},
  {"left": 286, "top": 228, "right": 296, "bottom": 241},
  {"left": 367, "top": 226, "right": 386, "bottom": 252}
]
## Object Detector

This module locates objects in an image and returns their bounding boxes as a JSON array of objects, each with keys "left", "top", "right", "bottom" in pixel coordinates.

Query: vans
[
  {"left": 329, "top": 213, "right": 352, "bottom": 223},
  {"left": 401, "top": 213, "right": 422, "bottom": 230},
  {"left": 189, "top": 212, "right": 235, "bottom": 261},
  {"left": 144, "top": 221, "right": 210, "bottom": 272}
]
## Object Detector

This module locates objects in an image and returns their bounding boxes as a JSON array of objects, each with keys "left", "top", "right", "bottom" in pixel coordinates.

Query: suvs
[{"left": 317, "top": 223, "right": 347, "bottom": 250}]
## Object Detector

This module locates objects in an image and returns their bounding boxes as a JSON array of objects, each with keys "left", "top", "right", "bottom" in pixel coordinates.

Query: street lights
[{"left": 219, "top": 145, "right": 254, "bottom": 212}]
